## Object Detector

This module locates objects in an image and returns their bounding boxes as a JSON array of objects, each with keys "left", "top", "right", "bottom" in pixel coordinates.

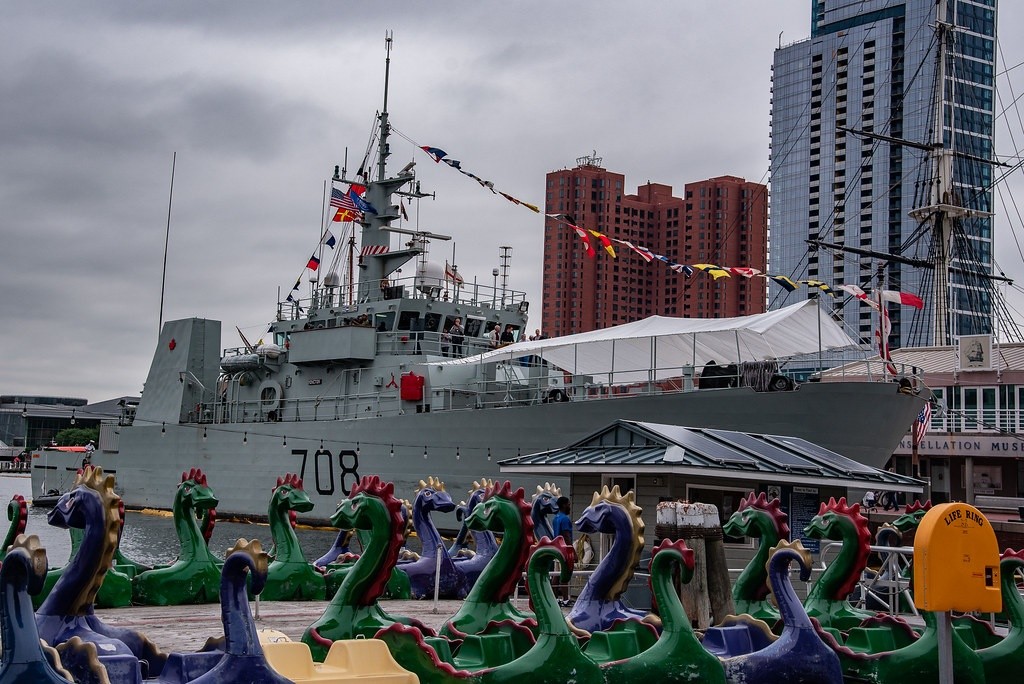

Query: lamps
[
  {"left": 429, "top": 286, "right": 443, "bottom": 301},
  {"left": 518, "top": 301, "right": 529, "bottom": 315}
]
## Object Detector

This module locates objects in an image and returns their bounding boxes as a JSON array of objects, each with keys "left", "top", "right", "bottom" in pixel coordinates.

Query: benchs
[{"left": 973, "top": 496, "right": 1024, "bottom": 513}]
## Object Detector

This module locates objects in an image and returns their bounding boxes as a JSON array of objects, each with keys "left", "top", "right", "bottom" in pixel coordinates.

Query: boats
[{"left": 30, "top": 27, "right": 937, "bottom": 535}]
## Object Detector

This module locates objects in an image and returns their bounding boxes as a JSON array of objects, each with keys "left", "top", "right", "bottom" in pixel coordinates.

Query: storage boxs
[
  {"left": 432, "top": 388, "right": 477, "bottom": 410},
  {"left": 573, "top": 374, "right": 699, "bottom": 401}
]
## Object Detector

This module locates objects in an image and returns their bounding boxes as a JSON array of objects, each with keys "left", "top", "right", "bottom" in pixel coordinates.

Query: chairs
[{"left": 97, "top": 563, "right": 1008, "bottom": 683}]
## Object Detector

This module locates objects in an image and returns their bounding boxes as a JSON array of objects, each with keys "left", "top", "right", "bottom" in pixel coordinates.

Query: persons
[
  {"left": 85, "top": 440, "right": 95, "bottom": 453},
  {"left": 883, "top": 468, "right": 900, "bottom": 512},
  {"left": 488, "top": 325, "right": 501, "bottom": 351},
  {"left": 441, "top": 328, "right": 451, "bottom": 357},
  {"left": 500, "top": 325, "right": 514, "bottom": 364},
  {"left": 359, "top": 313, "right": 371, "bottom": 327},
  {"left": 450, "top": 318, "right": 465, "bottom": 358},
  {"left": 520, "top": 328, "right": 545, "bottom": 367}
]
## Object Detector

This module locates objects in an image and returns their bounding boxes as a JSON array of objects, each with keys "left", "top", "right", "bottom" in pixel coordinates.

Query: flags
[
  {"left": 287, "top": 184, "right": 378, "bottom": 302},
  {"left": 875, "top": 307, "right": 897, "bottom": 377},
  {"left": 421, "top": 145, "right": 924, "bottom": 310},
  {"left": 917, "top": 402, "right": 931, "bottom": 444}
]
